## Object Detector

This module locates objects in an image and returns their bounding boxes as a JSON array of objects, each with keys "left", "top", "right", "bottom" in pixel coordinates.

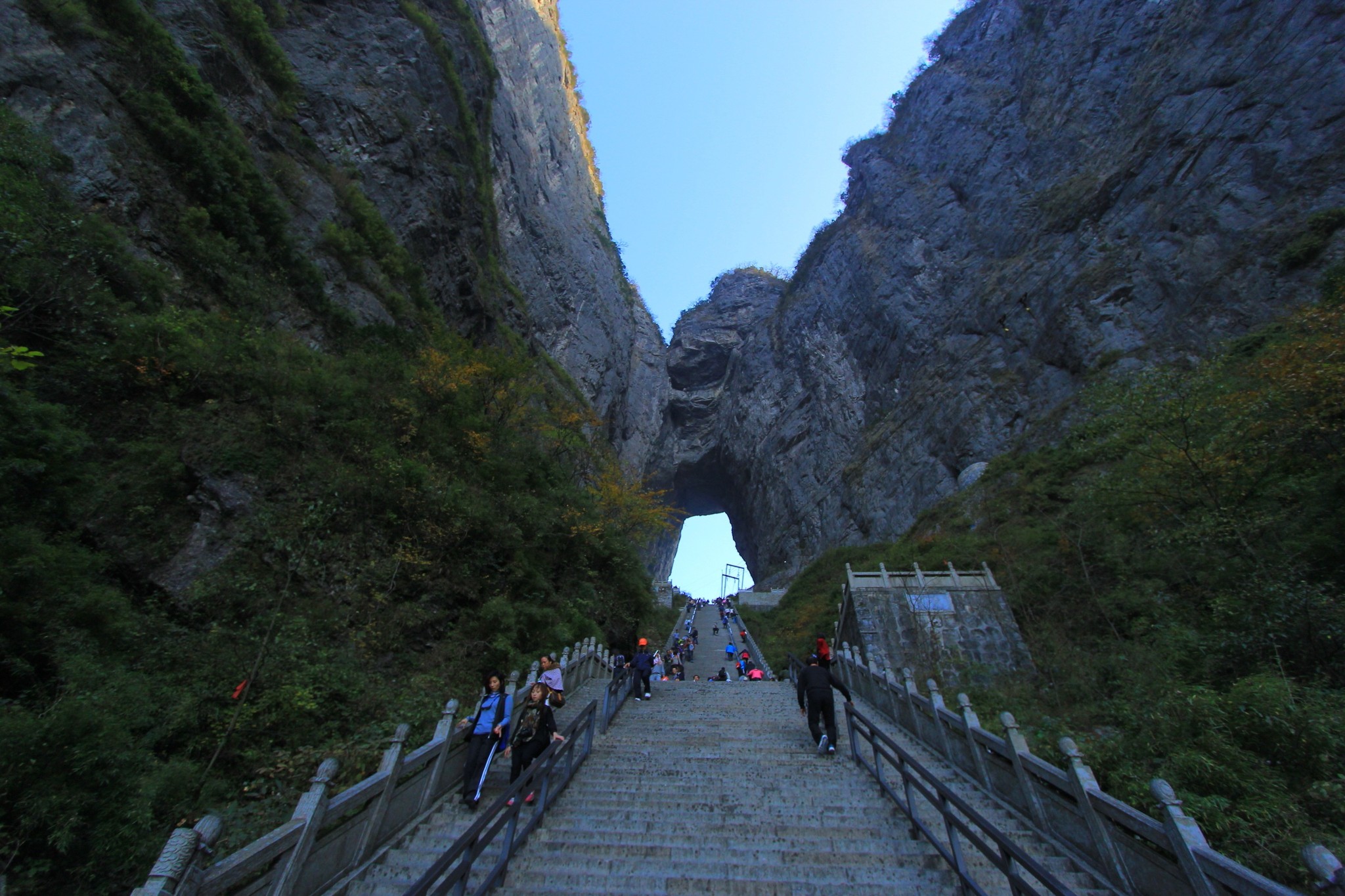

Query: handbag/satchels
[{"left": 549, "top": 692, "right": 566, "bottom": 709}]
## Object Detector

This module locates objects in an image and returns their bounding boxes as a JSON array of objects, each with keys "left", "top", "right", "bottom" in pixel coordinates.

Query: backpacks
[{"left": 615, "top": 654, "right": 626, "bottom": 667}]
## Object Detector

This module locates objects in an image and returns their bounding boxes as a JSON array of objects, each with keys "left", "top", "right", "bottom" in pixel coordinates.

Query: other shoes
[
  {"left": 817, "top": 734, "right": 835, "bottom": 755},
  {"left": 507, "top": 789, "right": 535, "bottom": 806},
  {"left": 635, "top": 692, "right": 651, "bottom": 701},
  {"left": 458, "top": 797, "right": 479, "bottom": 810}
]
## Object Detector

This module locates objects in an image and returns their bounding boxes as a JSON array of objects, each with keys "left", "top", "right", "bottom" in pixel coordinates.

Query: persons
[
  {"left": 621, "top": 637, "right": 659, "bottom": 701},
  {"left": 615, "top": 653, "right": 628, "bottom": 679},
  {"left": 529, "top": 653, "right": 564, "bottom": 713},
  {"left": 503, "top": 681, "right": 566, "bottom": 807},
  {"left": 796, "top": 656, "right": 854, "bottom": 754},
  {"left": 455, "top": 668, "right": 515, "bottom": 810},
  {"left": 814, "top": 631, "right": 831, "bottom": 670},
  {"left": 647, "top": 589, "right": 792, "bottom": 682}
]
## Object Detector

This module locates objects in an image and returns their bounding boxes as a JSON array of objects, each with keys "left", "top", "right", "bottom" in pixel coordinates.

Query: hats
[{"left": 638, "top": 638, "right": 648, "bottom": 646}]
